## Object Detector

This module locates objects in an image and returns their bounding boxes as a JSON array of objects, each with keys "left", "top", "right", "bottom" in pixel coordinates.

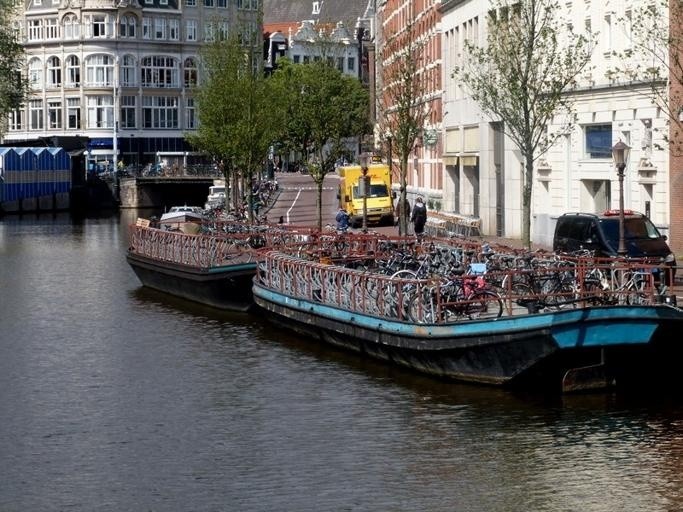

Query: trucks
[{"left": 336, "top": 163, "right": 397, "bottom": 229}]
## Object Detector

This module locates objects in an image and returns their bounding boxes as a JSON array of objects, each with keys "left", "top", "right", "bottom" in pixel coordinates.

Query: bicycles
[{"left": 140, "top": 160, "right": 664, "bottom": 325}]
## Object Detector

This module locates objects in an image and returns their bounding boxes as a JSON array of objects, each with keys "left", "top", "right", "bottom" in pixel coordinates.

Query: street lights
[{"left": 610, "top": 137, "right": 633, "bottom": 285}]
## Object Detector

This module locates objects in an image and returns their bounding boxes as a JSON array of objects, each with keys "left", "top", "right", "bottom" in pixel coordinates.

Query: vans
[{"left": 552, "top": 208, "right": 677, "bottom": 287}]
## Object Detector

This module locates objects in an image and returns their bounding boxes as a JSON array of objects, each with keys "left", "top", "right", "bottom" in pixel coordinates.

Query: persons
[
  {"left": 408, "top": 197, "right": 427, "bottom": 243},
  {"left": 334, "top": 207, "right": 350, "bottom": 234},
  {"left": 394, "top": 193, "right": 410, "bottom": 236},
  {"left": 245, "top": 188, "right": 264, "bottom": 219}
]
